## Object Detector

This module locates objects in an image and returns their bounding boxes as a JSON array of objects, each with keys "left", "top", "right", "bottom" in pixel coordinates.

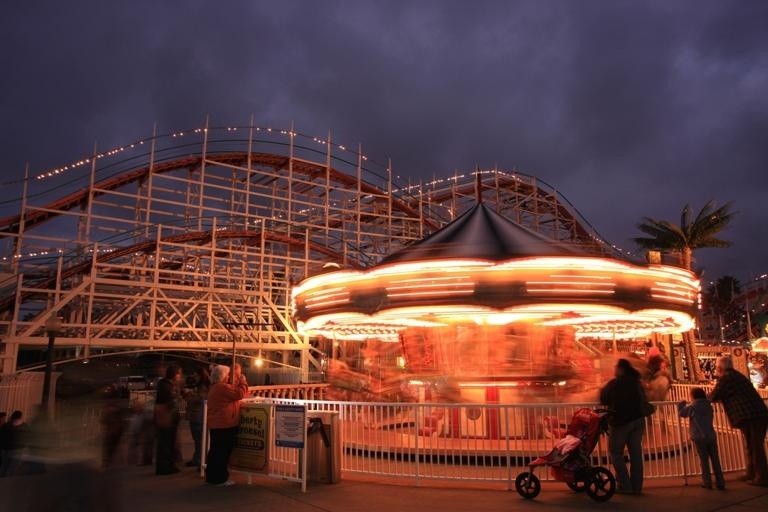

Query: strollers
[{"left": 514, "top": 408, "right": 616, "bottom": 502}]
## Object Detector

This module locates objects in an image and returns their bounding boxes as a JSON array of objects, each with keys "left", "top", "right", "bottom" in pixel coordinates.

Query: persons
[
  {"left": 0, "top": 412, "right": 6, "bottom": 428},
  {"left": 311, "top": 338, "right": 367, "bottom": 379},
  {"left": 94, "top": 355, "right": 248, "bottom": 488},
  {"left": 683, "top": 354, "right": 718, "bottom": 383},
  {"left": 708, "top": 358, "right": 767, "bottom": 487},
  {"left": 0, "top": 410, "right": 28, "bottom": 477},
  {"left": 599, "top": 359, "right": 654, "bottom": 495},
  {"left": 643, "top": 340, "right": 675, "bottom": 384},
  {"left": 677, "top": 388, "right": 726, "bottom": 490},
  {"left": 557, "top": 425, "right": 587, "bottom": 455}
]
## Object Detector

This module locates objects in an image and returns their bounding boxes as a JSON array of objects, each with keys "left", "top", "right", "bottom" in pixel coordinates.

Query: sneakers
[
  {"left": 213, "top": 479, "right": 236, "bottom": 487},
  {"left": 746, "top": 478, "right": 767, "bottom": 486},
  {"left": 185, "top": 460, "right": 196, "bottom": 466},
  {"left": 737, "top": 474, "right": 754, "bottom": 480}
]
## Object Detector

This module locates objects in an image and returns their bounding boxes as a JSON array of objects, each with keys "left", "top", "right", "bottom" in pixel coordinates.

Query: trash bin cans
[{"left": 299, "top": 408, "right": 343, "bottom": 485}]
